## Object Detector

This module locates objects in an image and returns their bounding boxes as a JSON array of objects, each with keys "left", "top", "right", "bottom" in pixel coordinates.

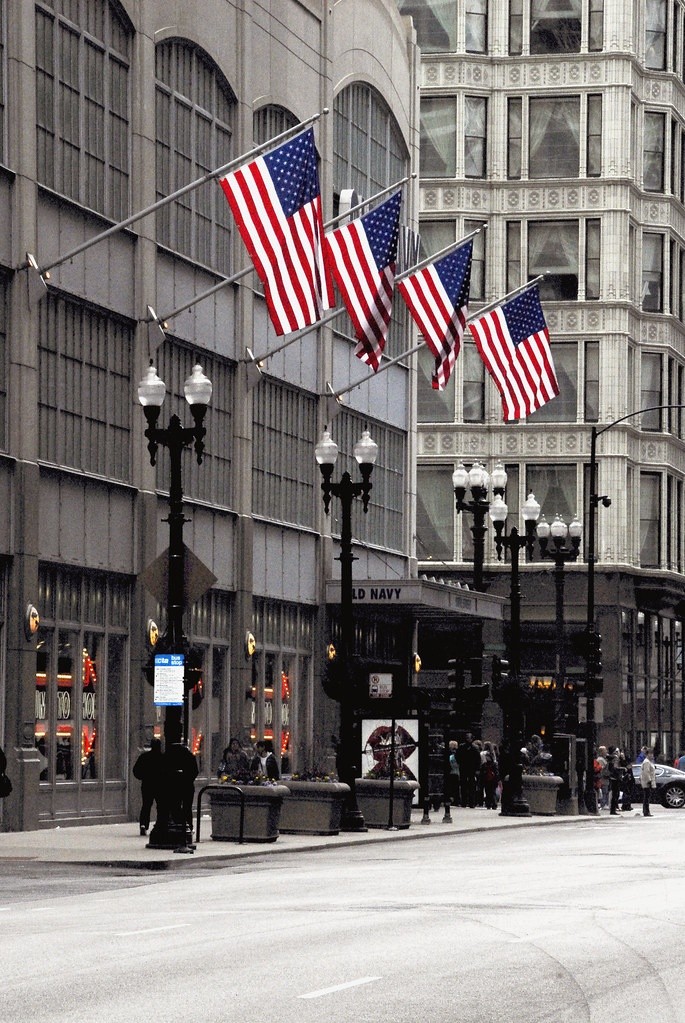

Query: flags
[
  {"left": 394, "top": 237, "right": 473, "bottom": 391},
  {"left": 322, "top": 188, "right": 404, "bottom": 374},
  {"left": 218, "top": 126, "right": 336, "bottom": 334},
  {"left": 464, "top": 285, "right": 560, "bottom": 424}
]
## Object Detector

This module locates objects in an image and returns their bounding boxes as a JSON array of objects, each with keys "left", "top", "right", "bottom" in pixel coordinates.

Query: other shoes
[
  {"left": 140, "top": 825, "right": 146, "bottom": 836},
  {"left": 611, "top": 812, "right": 619, "bottom": 815}
]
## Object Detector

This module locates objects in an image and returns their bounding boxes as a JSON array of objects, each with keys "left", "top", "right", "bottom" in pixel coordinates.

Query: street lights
[
  {"left": 619, "top": 612, "right": 646, "bottom": 763},
  {"left": 491, "top": 459, "right": 541, "bottom": 817},
  {"left": 452, "top": 456, "right": 491, "bottom": 738},
  {"left": 653, "top": 620, "right": 681, "bottom": 763},
  {"left": 536, "top": 513, "right": 584, "bottom": 815},
  {"left": 139, "top": 358, "right": 212, "bottom": 849},
  {"left": 314, "top": 423, "right": 379, "bottom": 828}
]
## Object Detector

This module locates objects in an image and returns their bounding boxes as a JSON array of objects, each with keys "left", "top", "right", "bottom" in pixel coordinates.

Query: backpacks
[{"left": 602, "top": 759, "right": 615, "bottom": 777}]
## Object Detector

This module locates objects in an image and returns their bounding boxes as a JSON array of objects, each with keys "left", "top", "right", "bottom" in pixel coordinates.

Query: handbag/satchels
[{"left": 0, "top": 772, "right": 13, "bottom": 798}]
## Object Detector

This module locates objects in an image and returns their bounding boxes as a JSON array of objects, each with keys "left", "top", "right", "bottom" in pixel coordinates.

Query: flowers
[
  {"left": 363, "top": 768, "right": 409, "bottom": 780},
  {"left": 218, "top": 767, "right": 278, "bottom": 787},
  {"left": 524, "top": 767, "right": 556, "bottom": 776},
  {"left": 283, "top": 767, "right": 339, "bottom": 783}
]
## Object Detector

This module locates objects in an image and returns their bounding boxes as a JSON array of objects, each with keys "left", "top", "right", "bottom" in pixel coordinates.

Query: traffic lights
[
  {"left": 566, "top": 681, "right": 578, "bottom": 714},
  {"left": 585, "top": 632, "right": 604, "bottom": 696},
  {"left": 446, "top": 658, "right": 458, "bottom": 730},
  {"left": 493, "top": 655, "right": 511, "bottom": 692}
]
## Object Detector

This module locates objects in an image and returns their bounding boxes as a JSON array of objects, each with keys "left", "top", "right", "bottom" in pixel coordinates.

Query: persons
[
  {"left": 438, "top": 733, "right": 509, "bottom": 810},
  {"left": 217, "top": 737, "right": 249, "bottom": 777},
  {"left": 250, "top": 739, "right": 279, "bottom": 782},
  {"left": 133, "top": 737, "right": 199, "bottom": 835},
  {"left": 520, "top": 735, "right": 551, "bottom": 770},
  {"left": 674, "top": 752, "right": 683, "bottom": 768},
  {"left": 82, "top": 752, "right": 96, "bottom": 779},
  {"left": 592, "top": 746, "right": 657, "bottom": 817}
]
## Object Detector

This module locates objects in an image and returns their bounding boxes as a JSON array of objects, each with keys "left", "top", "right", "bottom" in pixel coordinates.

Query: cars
[{"left": 619, "top": 764, "right": 685, "bottom": 808}]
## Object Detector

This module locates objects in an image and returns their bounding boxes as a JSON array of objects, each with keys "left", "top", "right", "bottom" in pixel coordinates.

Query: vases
[
  {"left": 505, "top": 774, "right": 564, "bottom": 816},
  {"left": 277, "top": 780, "right": 351, "bottom": 836},
  {"left": 205, "top": 782, "right": 291, "bottom": 843},
  {"left": 354, "top": 777, "right": 421, "bottom": 829}
]
